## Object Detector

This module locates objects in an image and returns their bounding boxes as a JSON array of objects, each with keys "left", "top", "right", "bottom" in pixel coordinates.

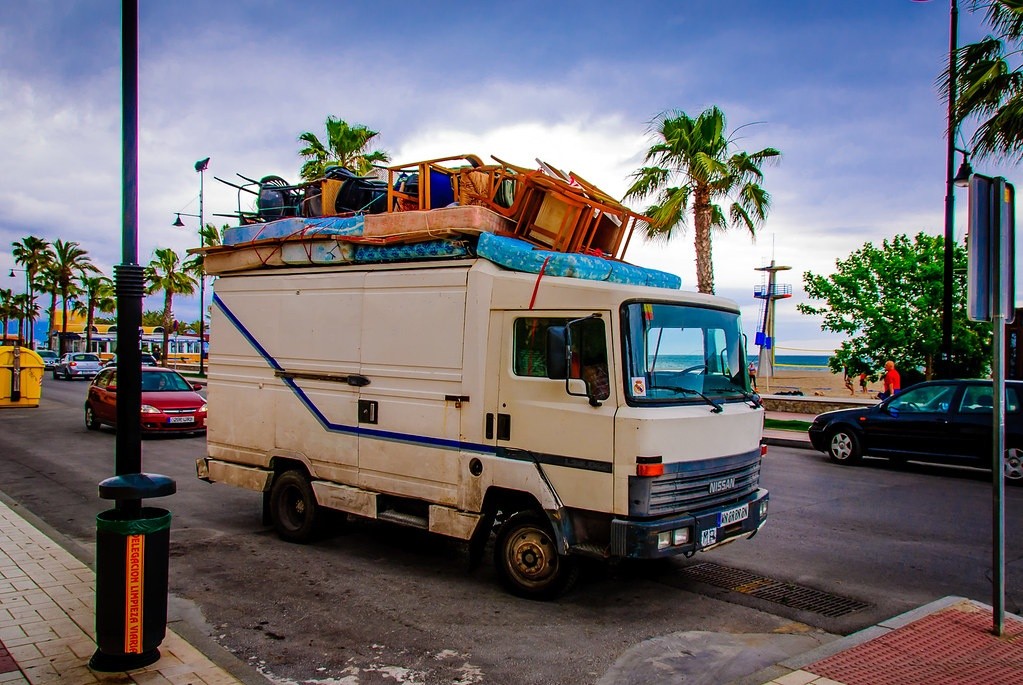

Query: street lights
[
  {"left": 935, "top": 0, "right": 978, "bottom": 377},
  {"left": 169, "top": 156, "right": 211, "bottom": 380},
  {"left": 8, "top": 267, "right": 31, "bottom": 348}
]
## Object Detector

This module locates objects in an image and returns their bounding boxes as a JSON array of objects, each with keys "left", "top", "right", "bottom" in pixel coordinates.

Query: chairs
[
  {"left": 214, "top": 154, "right": 659, "bottom": 267},
  {"left": 975, "top": 395, "right": 995, "bottom": 406}
]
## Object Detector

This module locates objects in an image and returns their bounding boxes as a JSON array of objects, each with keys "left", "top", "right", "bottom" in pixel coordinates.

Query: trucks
[{"left": 193, "top": 251, "right": 772, "bottom": 595}]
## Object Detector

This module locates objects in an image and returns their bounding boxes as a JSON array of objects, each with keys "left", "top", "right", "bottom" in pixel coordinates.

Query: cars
[
  {"left": 807, "top": 378, "right": 1023, "bottom": 486},
  {"left": 51, "top": 352, "right": 102, "bottom": 381},
  {"left": 35, "top": 350, "right": 59, "bottom": 370},
  {"left": 83, "top": 366, "right": 208, "bottom": 440},
  {"left": 105, "top": 351, "right": 158, "bottom": 368}
]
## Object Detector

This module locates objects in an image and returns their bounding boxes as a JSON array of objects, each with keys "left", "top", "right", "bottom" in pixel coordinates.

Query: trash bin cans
[{"left": 96, "top": 471, "right": 177, "bottom": 655}]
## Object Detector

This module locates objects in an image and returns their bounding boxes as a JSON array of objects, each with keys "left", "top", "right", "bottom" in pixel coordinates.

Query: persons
[
  {"left": 884, "top": 361, "right": 900, "bottom": 404},
  {"left": 842, "top": 362, "right": 854, "bottom": 395},
  {"left": 748, "top": 362, "right": 757, "bottom": 393},
  {"left": 582, "top": 342, "right": 628, "bottom": 400},
  {"left": 860, "top": 372, "right": 868, "bottom": 393}
]
